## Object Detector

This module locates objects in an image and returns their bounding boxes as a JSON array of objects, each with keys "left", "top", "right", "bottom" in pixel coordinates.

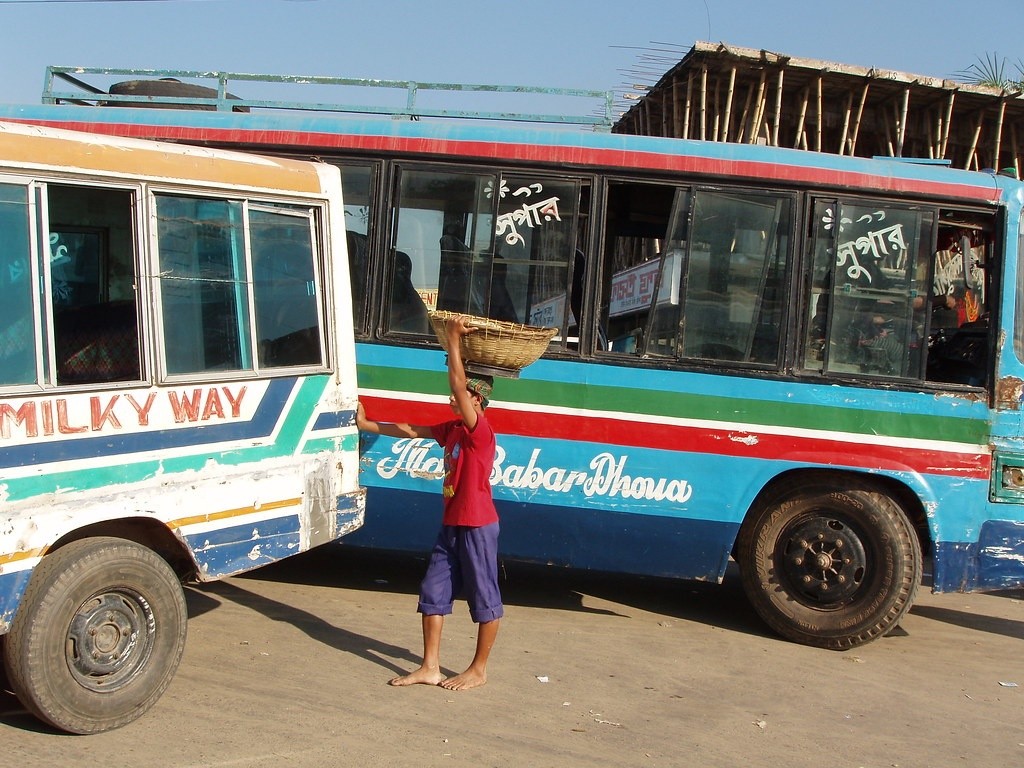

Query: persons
[
  {"left": 817, "top": 218, "right": 954, "bottom": 376},
  {"left": 355, "top": 314, "right": 503, "bottom": 691}
]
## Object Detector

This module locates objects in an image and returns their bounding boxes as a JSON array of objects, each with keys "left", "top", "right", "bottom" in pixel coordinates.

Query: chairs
[
  {"left": 433, "top": 234, "right": 484, "bottom": 335},
  {"left": 346, "top": 230, "right": 367, "bottom": 328},
  {"left": 389, "top": 249, "right": 429, "bottom": 333},
  {"left": 558, "top": 248, "right": 608, "bottom": 352},
  {"left": 478, "top": 250, "right": 520, "bottom": 324}
]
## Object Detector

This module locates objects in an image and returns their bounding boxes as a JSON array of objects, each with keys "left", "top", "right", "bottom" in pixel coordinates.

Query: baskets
[{"left": 425, "top": 308, "right": 558, "bottom": 370}]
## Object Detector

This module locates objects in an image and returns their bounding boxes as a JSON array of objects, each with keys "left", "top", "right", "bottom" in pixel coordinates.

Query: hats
[
  {"left": 870, "top": 316, "right": 893, "bottom": 326},
  {"left": 463, "top": 372, "right": 494, "bottom": 409}
]
